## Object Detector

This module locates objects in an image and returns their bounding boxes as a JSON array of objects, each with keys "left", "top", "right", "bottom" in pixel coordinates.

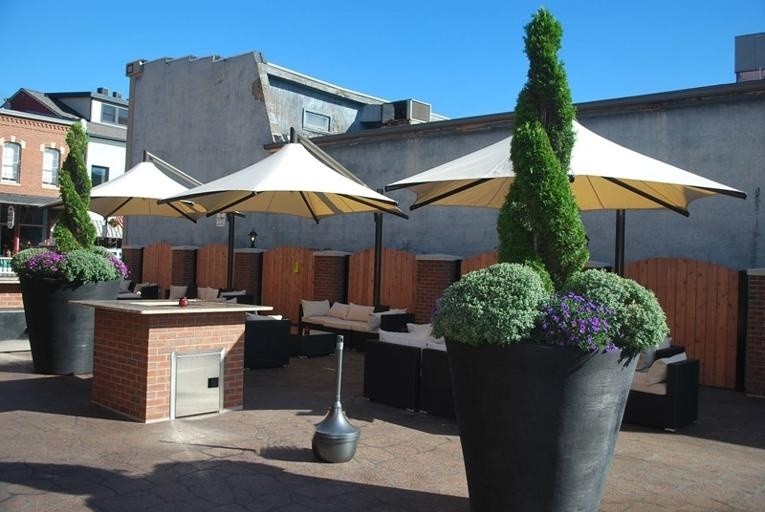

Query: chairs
[
  {"left": 622, "top": 344, "right": 700, "bottom": 432},
  {"left": 118, "top": 281, "right": 291, "bottom": 370},
  {"left": 364, "top": 340, "right": 454, "bottom": 419}
]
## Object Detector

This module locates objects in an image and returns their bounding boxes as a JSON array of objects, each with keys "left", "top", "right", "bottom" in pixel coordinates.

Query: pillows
[
  {"left": 301, "top": 299, "right": 409, "bottom": 331},
  {"left": 377, "top": 323, "right": 447, "bottom": 352},
  {"left": 636, "top": 336, "right": 686, "bottom": 385}
]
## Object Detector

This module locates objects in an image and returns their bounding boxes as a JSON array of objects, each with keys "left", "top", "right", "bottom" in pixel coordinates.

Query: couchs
[{"left": 298, "top": 302, "right": 415, "bottom": 352}]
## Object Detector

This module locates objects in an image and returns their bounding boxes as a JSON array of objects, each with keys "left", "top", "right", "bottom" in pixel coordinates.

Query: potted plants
[
  {"left": 431, "top": 8, "right": 670, "bottom": 512},
  {"left": 10, "top": 121, "right": 128, "bottom": 374}
]
